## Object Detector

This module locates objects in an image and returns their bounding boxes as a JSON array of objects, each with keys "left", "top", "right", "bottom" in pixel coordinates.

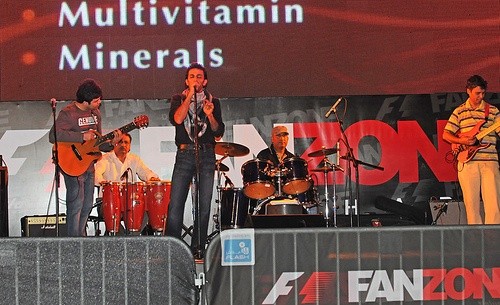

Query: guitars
[
  {"left": 52, "top": 114, "right": 148, "bottom": 177},
  {"left": 451, "top": 117, "right": 500, "bottom": 163}
]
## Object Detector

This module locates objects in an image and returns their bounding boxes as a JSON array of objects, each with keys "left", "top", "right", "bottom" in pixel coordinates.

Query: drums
[
  {"left": 221, "top": 187, "right": 258, "bottom": 228},
  {"left": 279, "top": 157, "right": 312, "bottom": 195},
  {"left": 241, "top": 158, "right": 276, "bottom": 200},
  {"left": 146, "top": 180, "right": 172, "bottom": 232},
  {"left": 100, "top": 181, "right": 121, "bottom": 233},
  {"left": 121, "top": 182, "right": 145, "bottom": 232}
]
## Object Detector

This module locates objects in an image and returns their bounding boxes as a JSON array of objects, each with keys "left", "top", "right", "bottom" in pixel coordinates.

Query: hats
[{"left": 272, "top": 126, "right": 289, "bottom": 136}]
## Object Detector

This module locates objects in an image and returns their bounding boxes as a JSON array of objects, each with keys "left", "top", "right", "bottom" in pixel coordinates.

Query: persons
[
  {"left": 164, "top": 63, "right": 225, "bottom": 259},
  {"left": 49, "top": 80, "right": 123, "bottom": 236},
  {"left": 442, "top": 74, "right": 500, "bottom": 225},
  {"left": 256, "top": 125, "right": 295, "bottom": 167},
  {"left": 94, "top": 132, "right": 161, "bottom": 236}
]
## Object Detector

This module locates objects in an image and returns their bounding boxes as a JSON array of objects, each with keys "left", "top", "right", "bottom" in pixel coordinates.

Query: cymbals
[
  {"left": 310, "top": 168, "right": 340, "bottom": 172},
  {"left": 308, "top": 148, "right": 340, "bottom": 157},
  {"left": 215, "top": 163, "right": 229, "bottom": 171},
  {"left": 214, "top": 142, "right": 249, "bottom": 156}
]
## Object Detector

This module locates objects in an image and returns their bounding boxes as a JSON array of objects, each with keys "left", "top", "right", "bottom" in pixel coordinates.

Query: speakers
[
  {"left": 85, "top": 218, "right": 106, "bottom": 236},
  {"left": 425, "top": 199, "right": 486, "bottom": 226},
  {"left": 21, "top": 215, "right": 68, "bottom": 238}
]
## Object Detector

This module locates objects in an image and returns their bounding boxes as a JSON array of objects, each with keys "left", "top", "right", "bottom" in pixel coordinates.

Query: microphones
[
  {"left": 51, "top": 98, "right": 57, "bottom": 112},
  {"left": 120, "top": 168, "right": 130, "bottom": 181},
  {"left": 325, "top": 97, "right": 343, "bottom": 118},
  {"left": 223, "top": 173, "right": 234, "bottom": 187},
  {"left": 336, "top": 141, "right": 340, "bottom": 166},
  {"left": 443, "top": 201, "right": 448, "bottom": 212}
]
